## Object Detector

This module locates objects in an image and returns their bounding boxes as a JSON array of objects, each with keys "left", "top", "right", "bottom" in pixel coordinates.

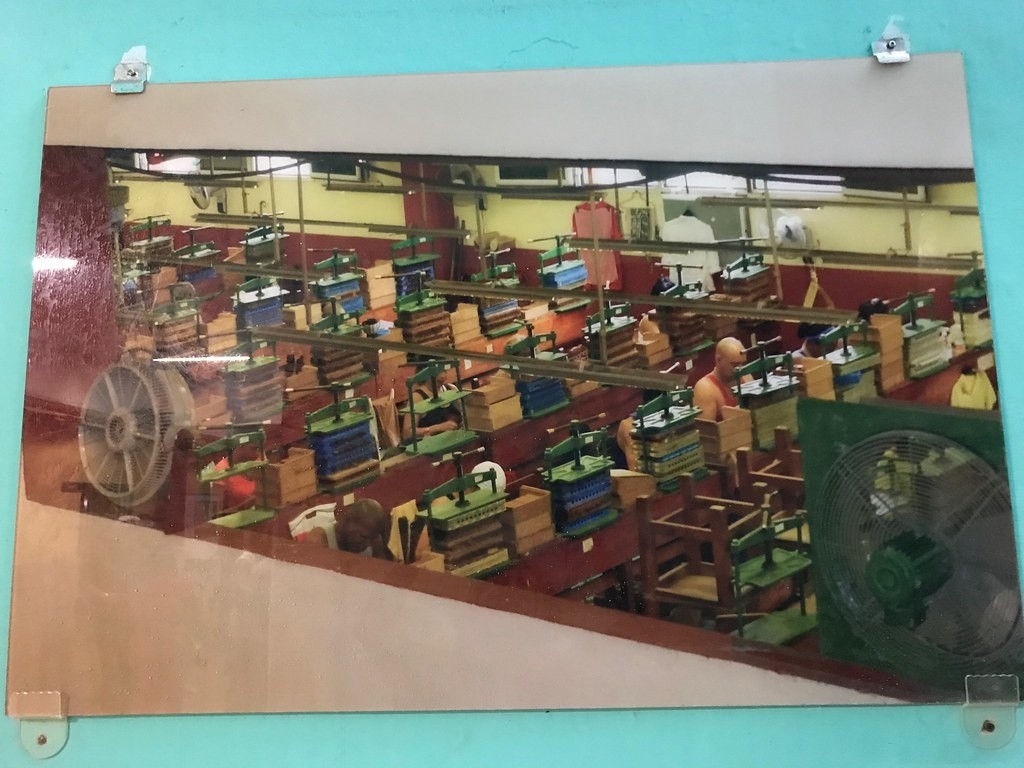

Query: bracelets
[{"left": 425, "top": 427, "right": 430, "bottom": 435}]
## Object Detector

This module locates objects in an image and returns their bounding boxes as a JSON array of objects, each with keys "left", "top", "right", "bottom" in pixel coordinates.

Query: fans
[
  {"left": 796, "top": 396, "right": 1023, "bottom": 700},
  {"left": 757, "top": 206, "right": 814, "bottom": 264},
  {"left": 187, "top": 170, "right": 227, "bottom": 213},
  {"left": 77, "top": 346, "right": 194, "bottom": 528}
]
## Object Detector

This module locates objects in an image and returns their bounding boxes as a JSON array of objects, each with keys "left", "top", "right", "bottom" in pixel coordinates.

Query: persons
[
  {"left": 637, "top": 277, "right": 675, "bottom": 342},
  {"left": 206, "top": 424, "right": 272, "bottom": 521},
  {"left": 692, "top": 336, "right": 755, "bottom": 423},
  {"left": 302, "top": 498, "right": 389, "bottom": 563},
  {"left": 790, "top": 323, "right": 841, "bottom": 360},
  {"left": 609, "top": 388, "right": 661, "bottom": 474},
  {"left": 402, "top": 353, "right": 461, "bottom": 442},
  {"left": 495, "top": 334, "right": 537, "bottom": 379}
]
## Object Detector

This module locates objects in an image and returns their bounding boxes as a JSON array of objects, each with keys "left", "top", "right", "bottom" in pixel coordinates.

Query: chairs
[
  {"left": 736, "top": 426, "right": 805, "bottom": 516},
  {"left": 635, "top": 471, "right": 772, "bottom": 620}
]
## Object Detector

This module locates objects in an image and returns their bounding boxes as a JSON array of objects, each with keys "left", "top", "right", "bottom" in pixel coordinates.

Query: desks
[{"left": 114, "top": 220, "right": 996, "bottom": 651}]
